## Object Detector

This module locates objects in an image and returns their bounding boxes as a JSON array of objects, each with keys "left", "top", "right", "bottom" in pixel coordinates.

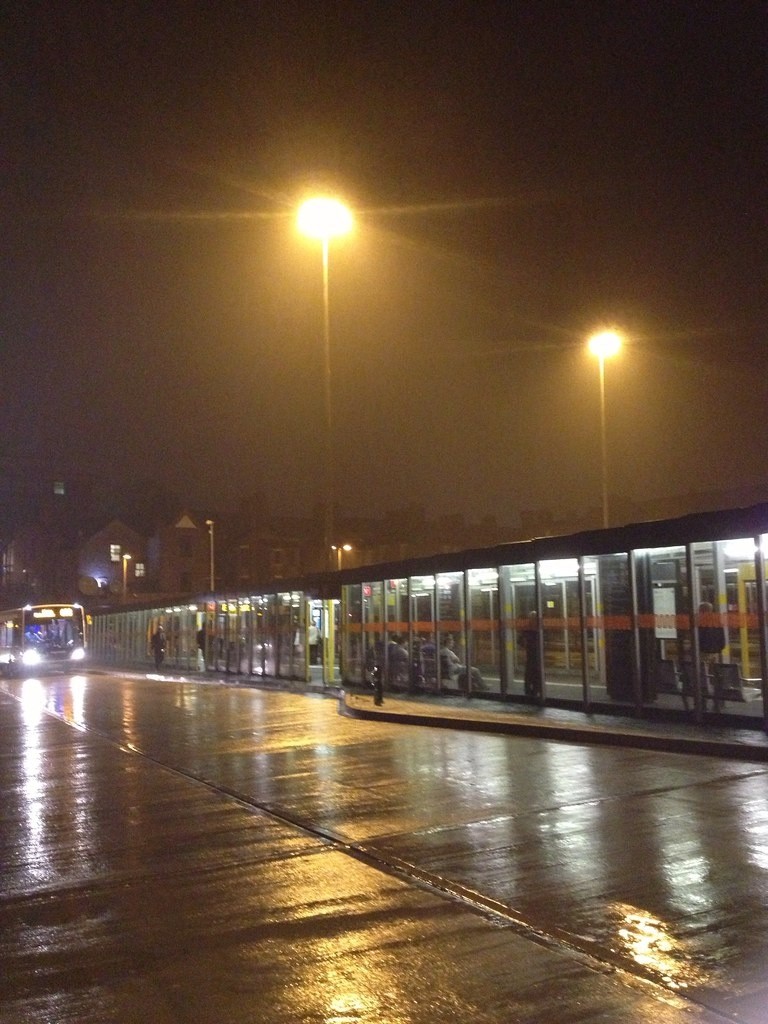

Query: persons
[
  {"left": 698, "top": 602, "right": 726, "bottom": 686},
  {"left": 25, "top": 625, "right": 45, "bottom": 644},
  {"left": 197, "top": 622, "right": 206, "bottom": 659},
  {"left": 293, "top": 619, "right": 320, "bottom": 665},
  {"left": 389, "top": 633, "right": 438, "bottom": 683},
  {"left": 438, "top": 638, "right": 492, "bottom": 689},
  {"left": 149, "top": 625, "right": 167, "bottom": 673},
  {"left": 518, "top": 611, "right": 545, "bottom": 697}
]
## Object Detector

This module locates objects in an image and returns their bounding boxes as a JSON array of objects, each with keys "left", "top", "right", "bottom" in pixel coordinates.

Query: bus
[{"left": 0, "top": 603, "right": 93, "bottom": 678}]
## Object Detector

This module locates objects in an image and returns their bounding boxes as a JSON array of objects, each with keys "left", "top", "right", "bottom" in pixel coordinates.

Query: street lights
[
  {"left": 593, "top": 331, "right": 623, "bottom": 527},
  {"left": 122, "top": 552, "right": 131, "bottom": 605},
  {"left": 206, "top": 520, "right": 216, "bottom": 594},
  {"left": 292, "top": 189, "right": 359, "bottom": 688}
]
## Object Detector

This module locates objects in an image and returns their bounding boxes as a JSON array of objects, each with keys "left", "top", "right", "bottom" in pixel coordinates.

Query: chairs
[
  {"left": 712, "top": 663, "right": 763, "bottom": 704},
  {"left": 646, "top": 659, "right": 681, "bottom": 696},
  {"left": 678, "top": 660, "right": 713, "bottom": 699},
  {"left": 366, "top": 647, "right": 453, "bottom": 680}
]
